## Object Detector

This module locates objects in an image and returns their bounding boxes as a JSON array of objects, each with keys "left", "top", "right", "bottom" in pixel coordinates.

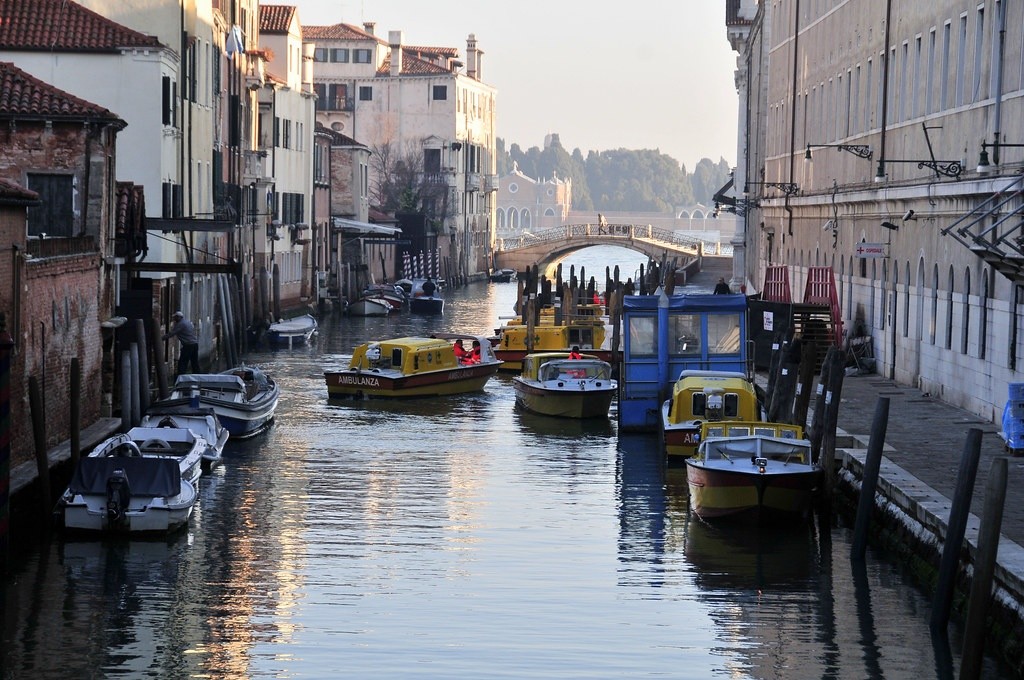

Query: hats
[{"left": 172, "top": 312, "right": 183, "bottom": 317}]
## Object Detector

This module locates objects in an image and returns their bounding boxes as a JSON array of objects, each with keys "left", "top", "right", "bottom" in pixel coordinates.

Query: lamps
[
  {"left": 712, "top": 202, "right": 746, "bottom": 217},
  {"left": 875, "top": 157, "right": 962, "bottom": 182},
  {"left": 976, "top": 139, "right": 1024, "bottom": 172},
  {"left": 881, "top": 222, "right": 899, "bottom": 230},
  {"left": 743, "top": 182, "right": 798, "bottom": 196},
  {"left": 804, "top": 141, "right": 873, "bottom": 162}
]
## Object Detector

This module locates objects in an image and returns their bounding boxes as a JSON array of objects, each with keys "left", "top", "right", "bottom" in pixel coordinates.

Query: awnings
[{"left": 336, "top": 217, "right": 402, "bottom": 234}]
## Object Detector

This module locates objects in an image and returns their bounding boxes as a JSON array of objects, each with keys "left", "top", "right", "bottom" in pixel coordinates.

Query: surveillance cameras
[
  {"left": 823, "top": 220, "right": 833, "bottom": 231},
  {"left": 902, "top": 210, "right": 914, "bottom": 221}
]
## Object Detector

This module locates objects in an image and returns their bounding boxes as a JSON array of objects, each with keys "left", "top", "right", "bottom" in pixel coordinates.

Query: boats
[
  {"left": 58, "top": 425, "right": 207, "bottom": 534},
  {"left": 267, "top": 313, "right": 318, "bottom": 345},
  {"left": 324, "top": 332, "right": 504, "bottom": 400},
  {"left": 125, "top": 406, "right": 231, "bottom": 476},
  {"left": 684, "top": 420, "right": 823, "bottom": 528},
  {"left": 145, "top": 361, "right": 281, "bottom": 441},
  {"left": 346, "top": 276, "right": 445, "bottom": 317},
  {"left": 486, "top": 261, "right": 606, "bottom": 372},
  {"left": 661, "top": 368, "right": 759, "bottom": 463},
  {"left": 511, "top": 352, "right": 618, "bottom": 420}
]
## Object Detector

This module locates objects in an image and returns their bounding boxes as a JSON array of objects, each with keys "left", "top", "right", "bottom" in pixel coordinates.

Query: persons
[
  {"left": 593, "top": 291, "right": 600, "bottom": 304},
  {"left": 162, "top": 311, "right": 200, "bottom": 374},
  {"left": 598, "top": 213, "right": 607, "bottom": 235},
  {"left": 454, "top": 340, "right": 480, "bottom": 363},
  {"left": 713, "top": 277, "right": 730, "bottom": 294},
  {"left": 422, "top": 277, "right": 435, "bottom": 297},
  {"left": 568, "top": 346, "right": 583, "bottom": 377}
]
